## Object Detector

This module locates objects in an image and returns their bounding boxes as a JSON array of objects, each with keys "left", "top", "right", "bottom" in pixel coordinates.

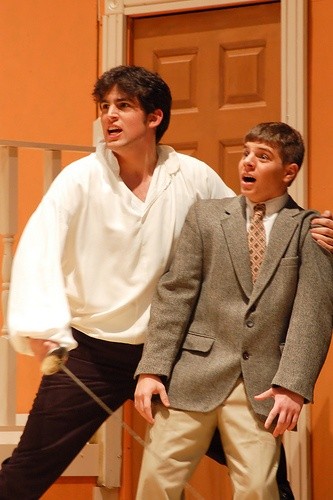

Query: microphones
[{"left": 40, "top": 345, "right": 69, "bottom": 375}]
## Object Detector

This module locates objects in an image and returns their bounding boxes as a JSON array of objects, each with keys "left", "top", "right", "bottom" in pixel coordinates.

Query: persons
[
  {"left": 126, "top": 121, "right": 332, "bottom": 499},
  {"left": 0, "top": 63, "right": 329, "bottom": 499}
]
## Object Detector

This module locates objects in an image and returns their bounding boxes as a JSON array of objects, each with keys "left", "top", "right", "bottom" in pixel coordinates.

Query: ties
[{"left": 249, "top": 205, "right": 269, "bottom": 289}]
